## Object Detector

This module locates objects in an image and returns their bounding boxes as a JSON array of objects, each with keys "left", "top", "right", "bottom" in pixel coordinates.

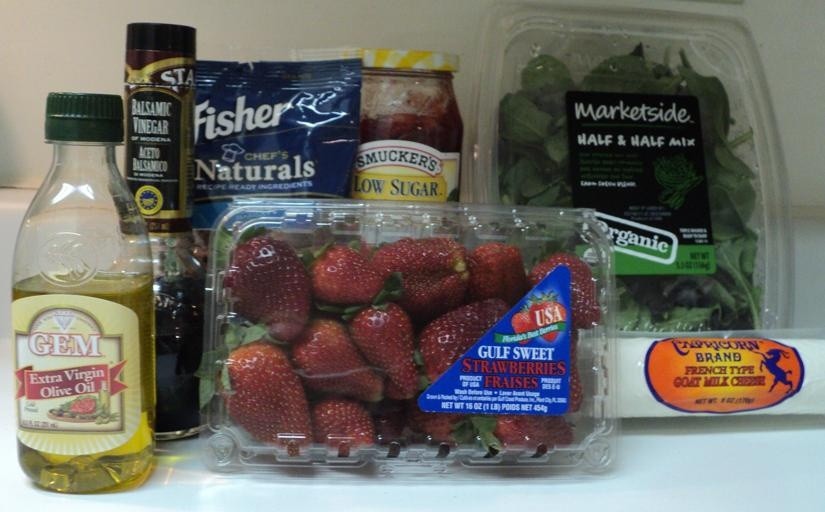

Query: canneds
[{"left": 342, "top": 47, "right": 465, "bottom": 201}]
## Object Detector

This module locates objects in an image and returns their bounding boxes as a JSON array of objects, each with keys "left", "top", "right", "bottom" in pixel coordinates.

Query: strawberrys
[{"left": 218, "top": 234, "right": 603, "bottom": 461}]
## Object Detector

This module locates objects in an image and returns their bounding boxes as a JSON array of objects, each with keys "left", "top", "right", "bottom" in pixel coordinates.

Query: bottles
[
  {"left": 124, "top": 22, "right": 209, "bottom": 444},
  {"left": 11, "top": 89, "right": 160, "bottom": 494}
]
